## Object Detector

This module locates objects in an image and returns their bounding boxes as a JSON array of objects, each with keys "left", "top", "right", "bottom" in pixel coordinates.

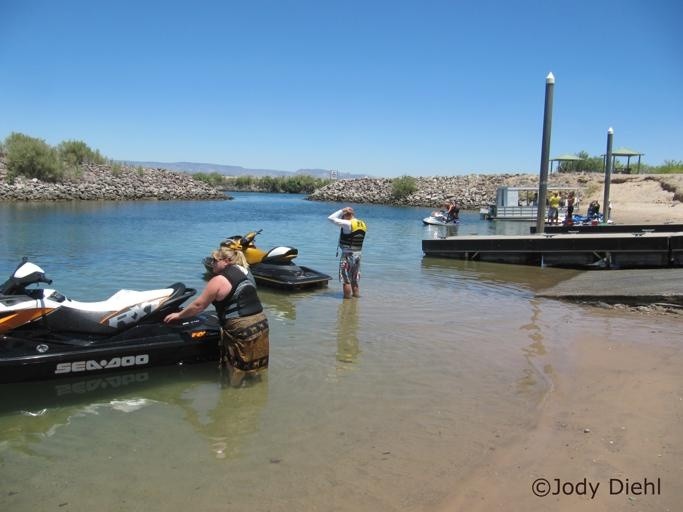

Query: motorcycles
[
  {"left": 422, "top": 210, "right": 461, "bottom": 226},
  {"left": 0, "top": 256, "right": 220, "bottom": 384},
  {"left": 201, "top": 229, "right": 333, "bottom": 290}
]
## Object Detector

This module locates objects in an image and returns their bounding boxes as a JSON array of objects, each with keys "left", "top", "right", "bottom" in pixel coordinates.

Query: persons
[
  {"left": 531, "top": 193, "right": 537, "bottom": 206},
  {"left": 547, "top": 190, "right": 611, "bottom": 225},
  {"left": 163, "top": 246, "right": 269, "bottom": 391},
  {"left": 328, "top": 206, "right": 366, "bottom": 297},
  {"left": 445, "top": 201, "right": 460, "bottom": 219}
]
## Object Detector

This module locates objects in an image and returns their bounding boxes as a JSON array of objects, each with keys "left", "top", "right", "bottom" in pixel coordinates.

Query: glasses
[{"left": 213, "top": 258, "right": 225, "bottom": 263}]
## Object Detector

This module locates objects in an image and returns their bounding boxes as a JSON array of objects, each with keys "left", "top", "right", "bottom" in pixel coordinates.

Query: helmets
[{"left": 341, "top": 207, "right": 353, "bottom": 219}]
